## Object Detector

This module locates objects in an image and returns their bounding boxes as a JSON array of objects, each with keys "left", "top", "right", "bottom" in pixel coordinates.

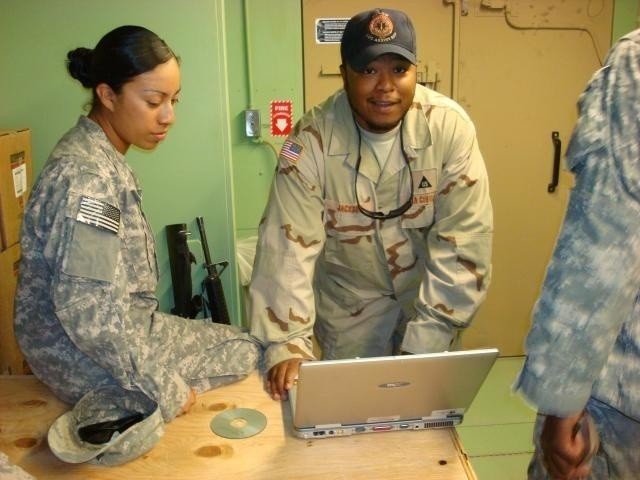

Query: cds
[{"left": 210, "top": 407, "right": 268, "bottom": 439}]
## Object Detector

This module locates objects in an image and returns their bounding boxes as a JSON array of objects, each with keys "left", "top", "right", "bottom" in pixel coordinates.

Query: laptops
[{"left": 287, "top": 347, "right": 500, "bottom": 440}]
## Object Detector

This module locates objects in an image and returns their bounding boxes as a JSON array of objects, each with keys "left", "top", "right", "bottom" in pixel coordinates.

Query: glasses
[{"left": 355, "top": 150, "right": 414, "bottom": 220}]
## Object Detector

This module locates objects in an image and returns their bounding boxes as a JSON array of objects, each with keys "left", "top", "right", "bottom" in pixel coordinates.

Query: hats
[
  {"left": 47, "top": 384, "right": 166, "bottom": 467},
  {"left": 340, "top": 7, "right": 419, "bottom": 74}
]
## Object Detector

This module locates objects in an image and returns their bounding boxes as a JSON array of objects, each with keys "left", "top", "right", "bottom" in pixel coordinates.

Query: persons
[
  {"left": 512, "top": 28, "right": 640, "bottom": 480},
  {"left": 249, "top": 7, "right": 494, "bottom": 402},
  {"left": 13, "top": 24, "right": 264, "bottom": 423}
]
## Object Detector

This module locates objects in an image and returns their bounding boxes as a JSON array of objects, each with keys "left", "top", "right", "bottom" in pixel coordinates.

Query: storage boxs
[{"left": 0, "top": 131, "right": 31, "bottom": 374}]
